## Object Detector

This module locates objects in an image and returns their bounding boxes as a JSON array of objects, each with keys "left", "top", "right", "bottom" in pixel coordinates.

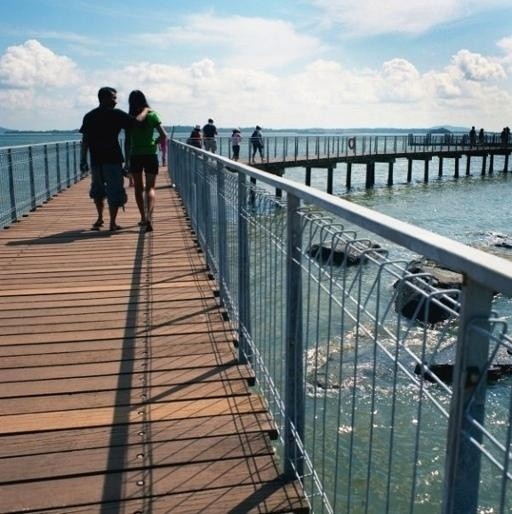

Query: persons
[
  {"left": 231, "top": 129, "right": 242, "bottom": 161},
  {"left": 79, "top": 87, "right": 154, "bottom": 231},
  {"left": 202, "top": 118, "right": 217, "bottom": 153},
  {"left": 190, "top": 124, "right": 202, "bottom": 147},
  {"left": 470, "top": 126, "right": 476, "bottom": 149},
  {"left": 159, "top": 137, "right": 168, "bottom": 166},
  {"left": 124, "top": 90, "right": 167, "bottom": 231},
  {"left": 120, "top": 159, "right": 135, "bottom": 186},
  {"left": 501, "top": 128, "right": 510, "bottom": 147},
  {"left": 251, "top": 125, "right": 266, "bottom": 162},
  {"left": 479, "top": 129, "right": 487, "bottom": 148}
]
